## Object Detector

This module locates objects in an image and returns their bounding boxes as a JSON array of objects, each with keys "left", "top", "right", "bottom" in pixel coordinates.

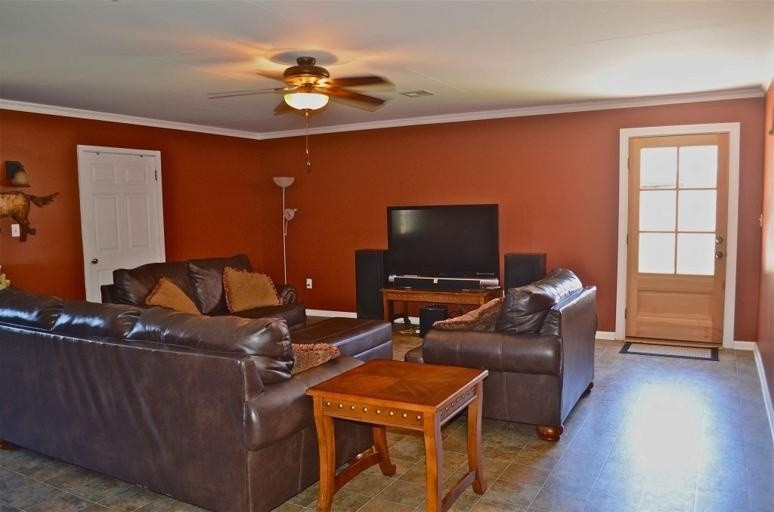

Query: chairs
[{"left": 404, "top": 268, "right": 597, "bottom": 441}]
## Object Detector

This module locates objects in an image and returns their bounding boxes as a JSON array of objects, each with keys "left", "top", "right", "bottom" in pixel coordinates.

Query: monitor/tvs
[{"left": 387, "top": 203, "right": 499, "bottom": 288}]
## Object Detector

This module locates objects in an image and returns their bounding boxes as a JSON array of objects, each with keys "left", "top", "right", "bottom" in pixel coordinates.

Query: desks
[
  {"left": 380, "top": 287, "right": 491, "bottom": 325},
  {"left": 307, "top": 359, "right": 490, "bottom": 512}
]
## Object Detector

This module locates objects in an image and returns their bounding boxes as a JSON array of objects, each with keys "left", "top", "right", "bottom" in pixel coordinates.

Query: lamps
[
  {"left": 273, "top": 177, "right": 298, "bottom": 285},
  {"left": 284, "top": 93, "right": 329, "bottom": 111}
]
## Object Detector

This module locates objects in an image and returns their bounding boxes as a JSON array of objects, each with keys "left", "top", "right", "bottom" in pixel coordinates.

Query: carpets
[{"left": 619, "top": 340, "right": 720, "bottom": 361}]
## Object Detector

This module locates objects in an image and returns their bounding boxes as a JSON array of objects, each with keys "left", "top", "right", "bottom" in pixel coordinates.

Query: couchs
[
  {"left": 0, "top": 293, "right": 374, "bottom": 512},
  {"left": 100, "top": 254, "right": 306, "bottom": 332}
]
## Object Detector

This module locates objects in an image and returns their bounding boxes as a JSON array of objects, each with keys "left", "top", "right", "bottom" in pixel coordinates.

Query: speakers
[
  {"left": 355, "top": 248, "right": 387, "bottom": 318},
  {"left": 419, "top": 305, "right": 447, "bottom": 337},
  {"left": 503, "top": 252, "right": 546, "bottom": 295}
]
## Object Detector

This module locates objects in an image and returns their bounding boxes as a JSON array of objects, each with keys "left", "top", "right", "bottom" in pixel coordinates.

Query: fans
[{"left": 207, "top": 57, "right": 396, "bottom": 172}]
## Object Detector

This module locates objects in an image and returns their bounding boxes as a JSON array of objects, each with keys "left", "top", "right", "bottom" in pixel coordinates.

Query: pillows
[
  {"left": 432, "top": 297, "right": 504, "bottom": 332},
  {"left": 189, "top": 264, "right": 224, "bottom": 314},
  {"left": 223, "top": 266, "right": 284, "bottom": 313},
  {"left": 291, "top": 343, "right": 340, "bottom": 374},
  {"left": 145, "top": 278, "right": 202, "bottom": 315}
]
